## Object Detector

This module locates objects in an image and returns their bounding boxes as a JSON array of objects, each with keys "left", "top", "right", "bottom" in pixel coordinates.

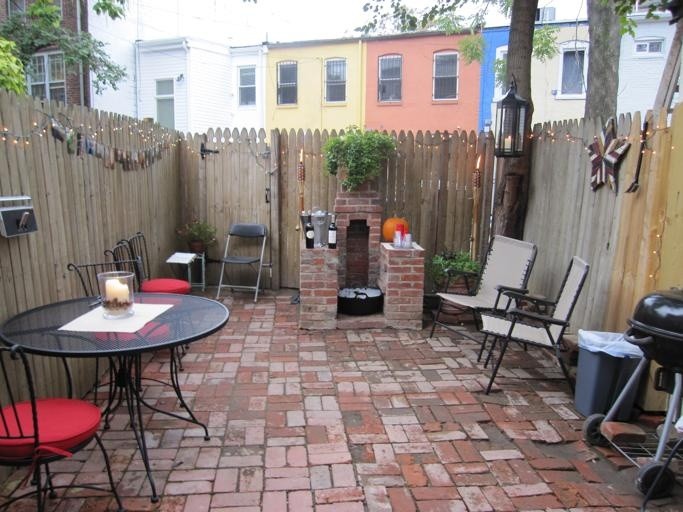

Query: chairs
[{"left": 429, "top": 234, "right": 590, "bottom": 395}]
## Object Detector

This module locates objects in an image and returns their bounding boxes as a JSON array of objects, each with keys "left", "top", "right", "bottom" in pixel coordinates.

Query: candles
[{"left": 297, "top": 148, "right": 305, "bottom": 181}]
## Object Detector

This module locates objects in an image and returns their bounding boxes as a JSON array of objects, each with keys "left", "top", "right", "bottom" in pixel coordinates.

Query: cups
[{"left": 96, "top": 270, "right": 135, "bottom": 321}]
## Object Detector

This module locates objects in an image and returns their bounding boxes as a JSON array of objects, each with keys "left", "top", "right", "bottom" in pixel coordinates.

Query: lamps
[{"left": 493, "top": 73, "right": 529, "bottom": 158}]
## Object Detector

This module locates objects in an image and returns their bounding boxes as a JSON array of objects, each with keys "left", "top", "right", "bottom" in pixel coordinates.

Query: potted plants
[{"left": 472, "top": 154, "right": 482, "bottom": 188}]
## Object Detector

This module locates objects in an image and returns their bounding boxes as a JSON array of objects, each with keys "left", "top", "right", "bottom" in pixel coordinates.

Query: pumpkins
[{"left": 382, "top": 214, "right": 409, "bottom": 242}]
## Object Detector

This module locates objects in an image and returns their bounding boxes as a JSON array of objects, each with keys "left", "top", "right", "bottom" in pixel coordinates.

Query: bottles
[
  {"left": 392, "top": 230, "right": 411, "bottom": 250},
  {"left": 304, "top": 215, "right": 314, "bottom": 249},
  {"left": 327, "top": 215, "right": 337, "bottom": 248}
]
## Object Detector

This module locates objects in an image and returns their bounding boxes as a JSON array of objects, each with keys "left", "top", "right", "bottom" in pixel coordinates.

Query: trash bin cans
[{"left": 574, "top": 329, "right": 644, "bottom": 421}]
